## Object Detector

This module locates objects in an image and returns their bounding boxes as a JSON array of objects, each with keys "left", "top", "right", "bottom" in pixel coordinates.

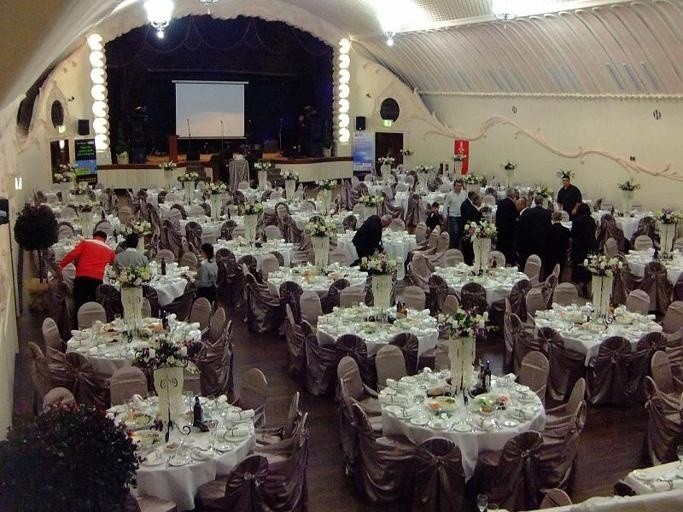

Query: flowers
[
  {"left": 450, "top": 151, "right": 468, "bottom": 162},
  {"left": 253, "top": 161, "right": 271, "bottom": 170},
  {"left": 500, "top": 159, "right": 519, "bottom": 170},
  {"left": 556, "top": 167, "right": 577, "bottom": 184},
  {"left": 157, "top": 160, "right": 177, "bottom": 169},
  {"left": 376, "top": 155, "right": 395, "bottom": 165},
  {"left": 400, "top": 144, "right": 415, "bottom": 156}
]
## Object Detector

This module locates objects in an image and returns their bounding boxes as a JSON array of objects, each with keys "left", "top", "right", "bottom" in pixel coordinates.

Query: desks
[
  {"left": 376, "top": 368, "right": 548, "bottom": 477},
  {"left": 315, "top": 305, "right": 440, "bottom": 365},
  {"left": 66, "top": 314, "right": 202, "bottom": 376},
  {"left": 431, "top": 264, "right": 529, "bottom": 308},
  {"left": 532, "top": 303, "right": 664, "bottom": 368},
  {"left": 266, "top": 263, "right": 369, "bottom": 303},
  {"left": 210, "top": 237, "right": 292, "bottom": 271},
  {"left": 336, "top": 229, "right": 417, "bottom": 277},
  {"left": 625, "top": 247, "right": 681, "bottom": 289},
  {"left": 99, "top": 262, "right": 195, "bottom": 306},
  {"left": 101, "top": 394, "right": 255, "bottom": 509},
  {"left": 50, "top": 234, "right": 131, "bottom": 278}
]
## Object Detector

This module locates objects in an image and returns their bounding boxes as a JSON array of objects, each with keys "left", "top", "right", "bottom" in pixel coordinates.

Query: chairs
[{"left": 23, "top": 169, "right": 681, "bottom": 511}]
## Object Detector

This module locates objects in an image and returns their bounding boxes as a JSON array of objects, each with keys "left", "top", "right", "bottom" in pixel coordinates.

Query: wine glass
[{"left": 676, "top": 446, "right": 683, "bottom": 468}]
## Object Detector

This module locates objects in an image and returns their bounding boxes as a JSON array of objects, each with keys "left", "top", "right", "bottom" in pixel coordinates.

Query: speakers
[
  {"left": 132, "top": 119, "right": 146, "bottom": 164},
  {"left": 356, "top": 116, "right": 365, "bottom": 130},
  {"left": 186, "top": 150, "right": 200, "bottom": 160},
  {"left": 78, "top": 119, "right": 89, "bottom": 135}
]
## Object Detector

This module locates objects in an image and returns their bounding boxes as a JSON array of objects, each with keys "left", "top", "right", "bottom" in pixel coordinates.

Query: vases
[
  {"left": 381, "top": 166, "right": 390, "bottom": 179},
  {"left": 403, "top": 155, "right": 411, "bottom": 172},
  {"left": 454, "top": 160, "right": 463, "bottom": 178}
]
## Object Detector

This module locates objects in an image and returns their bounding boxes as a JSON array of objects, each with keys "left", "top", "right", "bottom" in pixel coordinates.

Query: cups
[{"left": 322, "top": 150, "right": 331, "bottom": 158}]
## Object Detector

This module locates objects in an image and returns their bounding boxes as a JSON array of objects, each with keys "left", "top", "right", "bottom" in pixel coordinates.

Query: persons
[
  {"left": 442, "top": 180, "right": 465, "bottom": 248},
  {"left": 555, "top": 174, "right": 582, "bottom": 219},
  {"left": 109, "top": 234, "right": 152, "bottom": 273},
  {"left": 459, "top": 187, "right": 596, "bottom": 299},
  {"left": 183, "top": 243, "right": 217, "bottom": 305},
  {"left": 349, "top": 214, "right": 392, "bottom": 266},
  {"left": 56, "top": 228, "right": 113, "bottom": 323},
  {"left": 424, "top": 201, "right": 442, "bottom": 234}
]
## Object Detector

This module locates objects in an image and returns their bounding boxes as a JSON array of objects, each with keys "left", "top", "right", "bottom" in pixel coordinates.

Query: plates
[
  {"left": 633, "top": 468, "right": 654, "bottom": 482},
  {"left": 102, "top": 394, "right": 253, "bottom": 469},
  {"left": 50, "top": 212, "right": 194, "bottom": 292},
  {"left": 479, "top": 184, "right": 683, "bottom": 274},
  {"left": 65, "top": 322, "right": 202, "bottom": 361},
  {"left": 291, "top": 199, "right": 416, "bottom": 246},
  {"left": 147, "top": 186, "right": 290, "bottom": 262},
  {"left": 269, "top": 262, "right": 367, "bottom": 293},
  {"left": 429, "top": 260, "right": 519, "bottom": 296},
  {"left": 537, "top": 304, "right": 661, "bottom": 344},
  {"left": 315, "top": 302, "right": 438, "bottom": 346},
  {"left": 378, "top": 368, "right": 541, "bottom": 432}
]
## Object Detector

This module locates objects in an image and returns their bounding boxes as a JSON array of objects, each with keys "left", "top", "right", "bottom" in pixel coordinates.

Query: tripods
[{"left": 278, "top": 121, "right": 283, "bottom": 158}]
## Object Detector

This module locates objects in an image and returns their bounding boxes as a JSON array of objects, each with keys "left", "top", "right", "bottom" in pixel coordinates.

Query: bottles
[{"left": 436, "top": 162, "right": 450, "bottom": 180}]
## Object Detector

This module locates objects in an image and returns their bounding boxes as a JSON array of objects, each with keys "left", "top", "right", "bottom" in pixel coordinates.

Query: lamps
[
  {"left": 89, "top": 31, "right": 109, "bottom": 153},
  {"left": 384, "top": 30, "right": 395, "bottom": 47},
  {"left": 141, "top": 1, "right": 176, "bottom": 41},
  {"left": 332, "top": 35, "right": 352, "bottom": 145}
]
